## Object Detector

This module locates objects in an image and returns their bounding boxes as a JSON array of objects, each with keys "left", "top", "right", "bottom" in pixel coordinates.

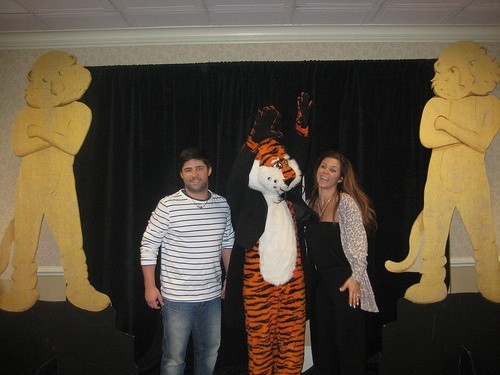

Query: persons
[
  {"left": 301, "top": 153, "right": 381, "bottom": 375},
  {"left": 139, "top": 148, "right": 236, "bottom": 375}
]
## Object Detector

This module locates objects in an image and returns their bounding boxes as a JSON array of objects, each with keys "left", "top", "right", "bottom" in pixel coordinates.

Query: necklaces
[
  {"left": 190, "top": 198, "right": 209, "bottom": 209},
  {"left": 316, "top": 190, "right": 338, "bottom": 217}
]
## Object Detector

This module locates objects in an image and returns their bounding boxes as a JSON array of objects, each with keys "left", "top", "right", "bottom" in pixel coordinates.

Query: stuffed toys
[{"left": 231, "top": 90, "right": 314, "bottom": 375}]
